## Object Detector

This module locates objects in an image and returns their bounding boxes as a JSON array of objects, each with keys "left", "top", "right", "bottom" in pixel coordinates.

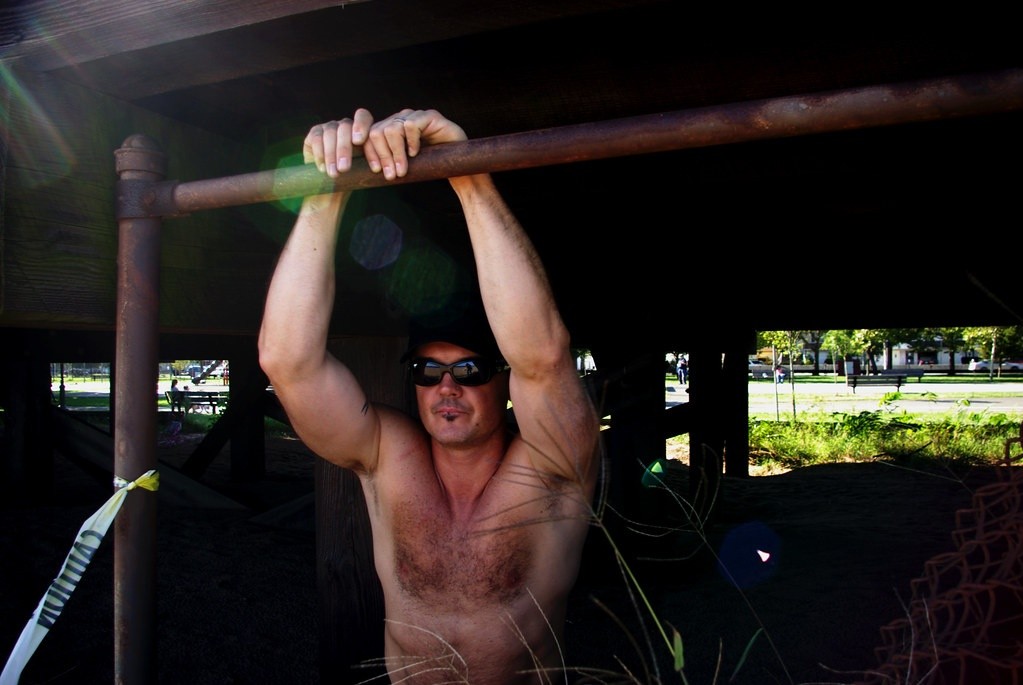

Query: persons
[
  {"left": 171, "top": 380, "right": 180, "bottom": 392},
  {"left": 676, "top": 353, "right": 688, "bottom": 385},
  {"left": 256, "top": 103, "right": 597, "bottom": 685},
  {"left": 775, "top": 365, "right": 786, "bottom": 383}
]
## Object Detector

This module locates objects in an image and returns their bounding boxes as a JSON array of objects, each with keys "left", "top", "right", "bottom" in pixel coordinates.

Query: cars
[
  {"left": 748, "top": 346, "right": 790, "bottom": 378},
  {"left": 967, "top": 358, "right": 1023, "bottom": 373},
  {"left": 159, "top": 363, "right": 229, "bottom": 378},
  {"left": 50, "top": 367, "right": 110, "bottom": 377}
]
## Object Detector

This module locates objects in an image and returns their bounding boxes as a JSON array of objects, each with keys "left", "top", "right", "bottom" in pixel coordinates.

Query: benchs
[
  {"left": 754, "top": 369, "right": 791, "bottom": 383},
  {"left": 847, "top": 374, "right": 908, "bottom": 394},
  {"left": 882, "top": 368, "right": 925, "bottom": 383},
  {"left": 165, "top": 390, "right": 220, "bottom": 415}
]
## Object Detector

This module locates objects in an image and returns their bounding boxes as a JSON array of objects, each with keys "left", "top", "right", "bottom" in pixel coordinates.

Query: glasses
[{"left": 409, "top": 356, "right": 511, "bottom": 387}]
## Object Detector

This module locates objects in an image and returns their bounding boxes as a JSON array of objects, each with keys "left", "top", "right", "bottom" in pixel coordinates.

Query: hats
[{"left": 400, "top": 303, "right": 498, "bottom": 363}]
